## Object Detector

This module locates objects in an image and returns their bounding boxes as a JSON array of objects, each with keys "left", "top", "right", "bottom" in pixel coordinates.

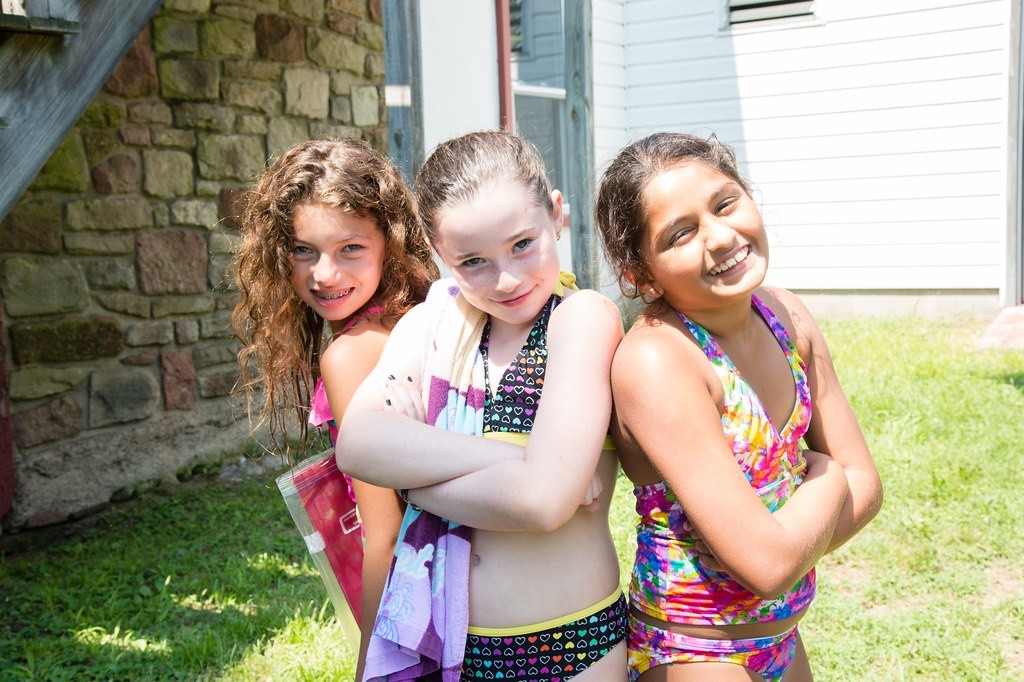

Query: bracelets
[{"left": 398, "top": 489, "right": 422, "bottom": 512}]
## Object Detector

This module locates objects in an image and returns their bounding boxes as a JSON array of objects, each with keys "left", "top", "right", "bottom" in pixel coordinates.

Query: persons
[
  {"left": 335, "top": 131, "right": 626, "bottom": 682},
  {"left": 230, "top": 139, "right": 440, "bottom": 681},
  {"left": 594, "top": 132, "right": 885, "bottom": 681}
]
